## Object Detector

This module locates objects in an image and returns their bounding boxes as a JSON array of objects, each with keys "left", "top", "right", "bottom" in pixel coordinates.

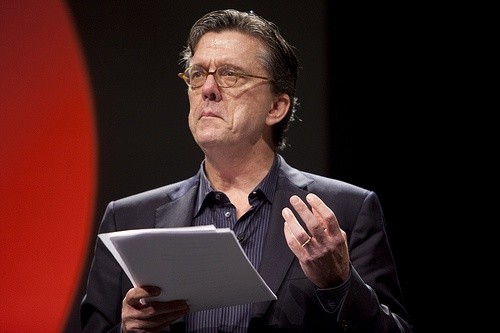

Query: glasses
[{"left": 179, "top": 66, "right": 274, "bottom": 88}]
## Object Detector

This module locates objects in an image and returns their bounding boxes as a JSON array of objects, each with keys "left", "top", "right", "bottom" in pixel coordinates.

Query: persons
[{"left": 78, "top": 9, "right": 412, "bottom": 333}]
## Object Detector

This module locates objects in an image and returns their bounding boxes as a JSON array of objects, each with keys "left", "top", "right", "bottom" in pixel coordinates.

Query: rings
[{"left": 300, "top": 237, "right": 312, "bottom": 247}]
